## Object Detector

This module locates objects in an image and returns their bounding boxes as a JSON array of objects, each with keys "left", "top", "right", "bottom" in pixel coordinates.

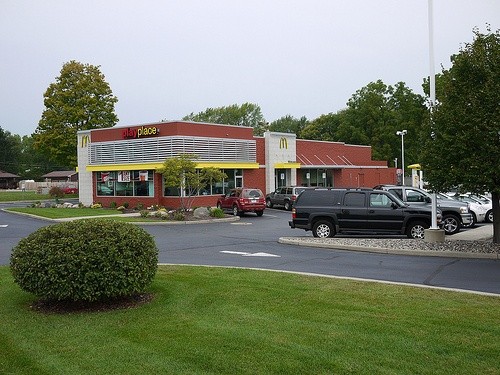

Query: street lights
[{"left": 396, "top": 130, "right": 407, "bottom": 185}]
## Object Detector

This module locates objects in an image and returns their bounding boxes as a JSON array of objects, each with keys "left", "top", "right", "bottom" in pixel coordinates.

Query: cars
[
  {"left": 406, "top": 190, "right": 493, "bottom": 229},
  {"left": 59, "top": 183, "right": 78, "bottom": 195}
]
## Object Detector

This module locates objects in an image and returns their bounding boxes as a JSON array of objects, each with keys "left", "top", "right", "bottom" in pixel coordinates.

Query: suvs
[
  {"left": 370, "top": 184, "right": 473, "bottom": 236},
  {"left": 216, "top": 188, "right": 266, "bottom": 217},
  {"left": 265, "top": 186, "right": 309, "bottom": 211},
  {"left": 288, "top": 187, "right": 443, "bottom": 240}
]
